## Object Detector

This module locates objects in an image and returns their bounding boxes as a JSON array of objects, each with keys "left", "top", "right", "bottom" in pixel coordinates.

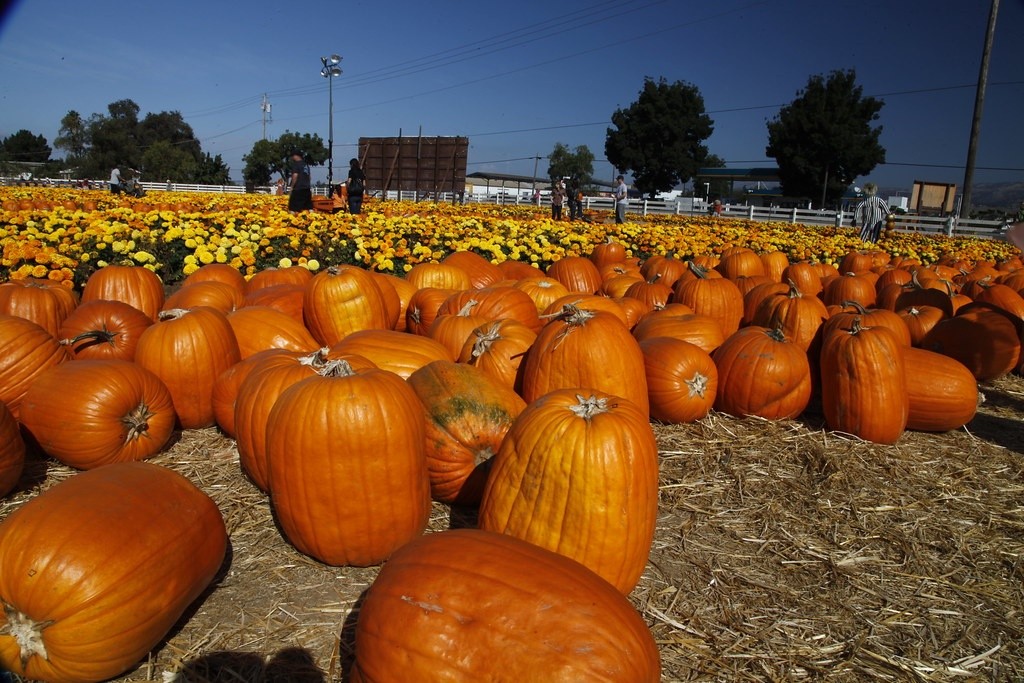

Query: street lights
[
  {"left": 704, "top": 183, "right": 710, "bottom": 203},
  {"left": 319, "top": 53, "right": 343, "bottom": 197}
]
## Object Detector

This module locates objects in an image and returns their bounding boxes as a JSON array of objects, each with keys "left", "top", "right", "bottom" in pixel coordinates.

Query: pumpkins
[
  {"left": 3, "top": 198, "right": 238, "bottom": 211},
  {"left": 0, "top": 233, "right": 1024, "bottom": 683}
]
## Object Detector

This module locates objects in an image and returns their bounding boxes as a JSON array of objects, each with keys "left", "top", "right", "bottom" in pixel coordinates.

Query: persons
[
  {"left": 534, "top": 190, "right": 540, "bottom": 205},
  {"left": 328, "top": 158, "right": 365, "bottom": 214},
  {"left": 457, "top": 192, "right": 466, "bottom": 206},
  {"left": 943, "top": 209, "right": 956, "bottom": 236},
  {"left": 713, "top": 200, "right": 721, "bottom": 217},
  {"left": 288, "top": 152, "right": 314, "bottom": 215},
  {"left": 68, "top": 178, "right": 95, "bottom": 190},
  {"left": 550, "top": 182, "right": 583, "bottom": 222},
  {"left": 616, "top": 175, "right": 627, "bottom": 224},
  {"left": 424, "top": 192, "right": 430, "bottom": 201},
  {"left": 20, "top": 175, "right": 50, "bottom": 186},
  {"left": 109, "top": 166, "right": 127, "bottom": 196},
  {"left": 852, "top": 184, "right": 891, "bottom": 244},
  {"left": 277, "top": 179, "right": 283, "bottom": 195}
]
